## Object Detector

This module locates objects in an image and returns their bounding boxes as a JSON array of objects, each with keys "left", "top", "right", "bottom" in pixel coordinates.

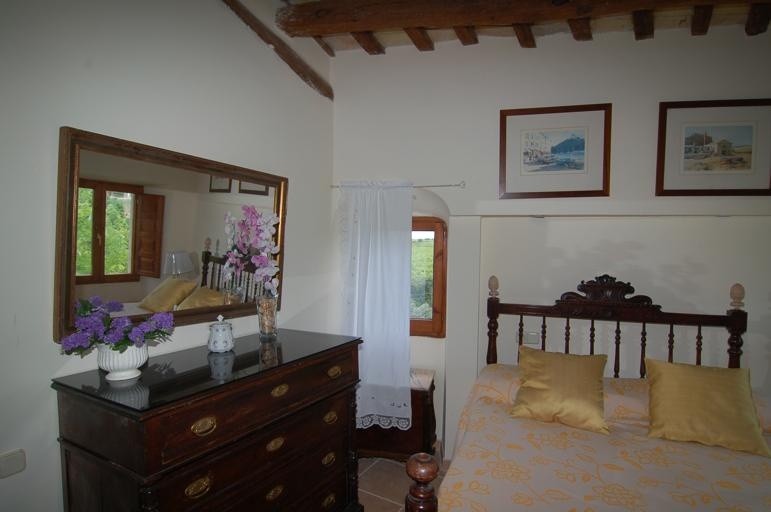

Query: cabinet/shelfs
[{"left": 52, "top": 326, "right": 366, "bottom": 511}]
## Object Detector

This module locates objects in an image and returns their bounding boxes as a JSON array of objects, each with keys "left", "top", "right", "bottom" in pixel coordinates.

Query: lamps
[{"left": 163, "top": 249, "right": 198, "bottom": 279}]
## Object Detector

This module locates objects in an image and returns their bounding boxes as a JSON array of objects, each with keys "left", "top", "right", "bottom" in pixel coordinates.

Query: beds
[{"left": 395, "top": 267, "right": 771, "bottom": 511}]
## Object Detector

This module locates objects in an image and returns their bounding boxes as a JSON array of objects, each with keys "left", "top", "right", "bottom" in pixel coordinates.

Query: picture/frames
[
  {"left": 653, "top": 97, "right": 771, "bottom": 201},
  {"left": 237, "top": 180, "right": 270, "bottom": 196},
  {"left": 497, "top": 100, "right": 614, "bottom": 200},
  {"left": 208, "top": 173, "right": 233, "bottom": 193}
]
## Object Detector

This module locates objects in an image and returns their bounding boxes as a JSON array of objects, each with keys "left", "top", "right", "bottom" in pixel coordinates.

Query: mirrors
[{"left": 50, "top": 125, "right": 291, "bottom": 343}]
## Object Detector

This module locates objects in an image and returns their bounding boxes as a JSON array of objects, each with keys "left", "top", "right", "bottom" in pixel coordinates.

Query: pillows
[
  {"left": 513, "top": 341, "right": 616, "bottom": 436},
  {"left": 640, "top": 355, "right": 770, "bottom": 462},
  {"left": 178, "top": 284, "right": 229, "bottom": 313},
  {"left": 136, "top": 276, "right": 198, "bottom": 315}
]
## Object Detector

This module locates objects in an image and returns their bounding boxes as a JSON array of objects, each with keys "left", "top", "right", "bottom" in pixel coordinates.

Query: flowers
[
  {"left": 237, "top": 204, "right": 284, "bottom": 299},
  {"left": 60, "top": 309, "right": 178, "bottom": 357},
  {"left": 76, "top": 290, "right": 124, "bottom": 315},
  {"left": 218, "top": 207, "right": 247, "bottom": 288}
]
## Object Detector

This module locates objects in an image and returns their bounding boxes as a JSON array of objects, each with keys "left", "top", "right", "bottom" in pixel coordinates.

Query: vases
[
  {"left": 92, "top": 339, "right": 152, "bottom": 381},
  {"left": 255, "top": 294, "right": 281, "bottom": 339},
  {"left": 227, "top": 286, "right": 244, "bottom": 307}
]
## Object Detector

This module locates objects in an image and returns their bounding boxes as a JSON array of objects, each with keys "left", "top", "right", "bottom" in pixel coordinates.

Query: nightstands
[{"left": 356, "top": 365, "right": 439, "bottom": 465}]
